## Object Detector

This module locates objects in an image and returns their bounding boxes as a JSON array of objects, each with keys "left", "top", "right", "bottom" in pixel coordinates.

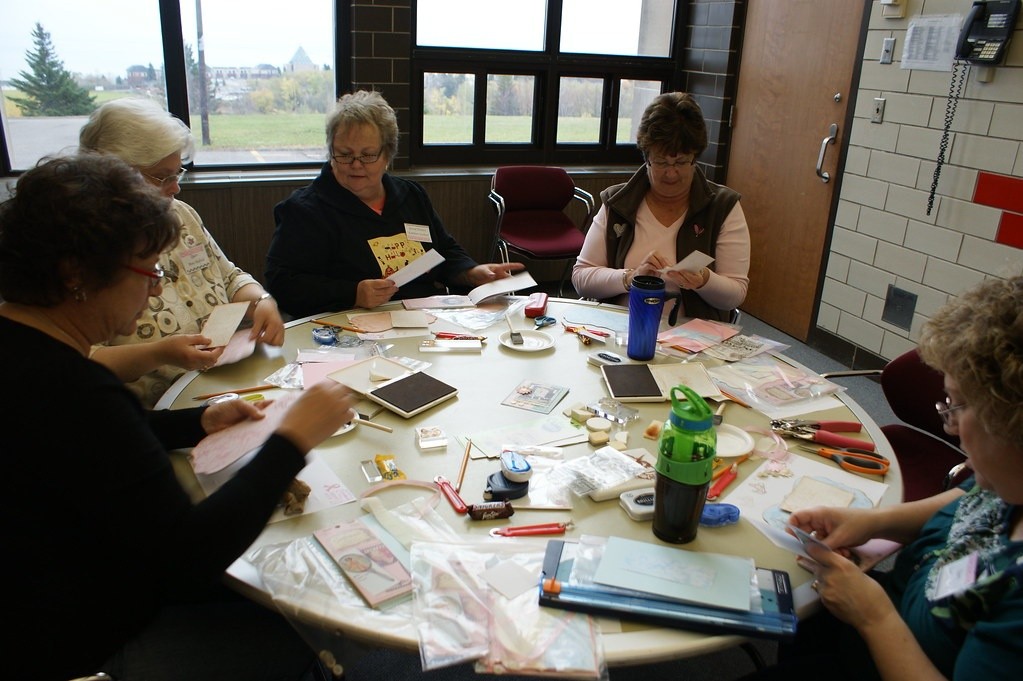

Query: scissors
[
  {"left": 799, "top": 445, "right": 891, "bottom": 477},
  {"left": 322, "top": 325, "right": 343, "bottom": 342},
  {"left": 534, "top": 316, "right": 557, "bottom": 330}
]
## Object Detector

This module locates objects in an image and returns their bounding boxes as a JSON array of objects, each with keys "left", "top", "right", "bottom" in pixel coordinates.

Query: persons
[
  {"left": 81, "top": 97, "right": 284, "bottom": 404},
  {"left": 572, "top": 93, "right": 751, "bottom": 324},
  {"left": 262, "top": 91, "right": 523, "bottom": 320},
  {"left": 0, "top": 153, "right": 357, "bottom": 681},
  {"left": 786, "top": 275, "right": 1023, "bottom": 681}
]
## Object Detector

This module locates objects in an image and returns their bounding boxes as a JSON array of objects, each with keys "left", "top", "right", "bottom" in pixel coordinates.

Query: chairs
[
  {"left": 820, "top": 344, "right": 974, "bottom": 503},
  {"left": 488, "top": 167, "right": 595, "bottom": 298}
]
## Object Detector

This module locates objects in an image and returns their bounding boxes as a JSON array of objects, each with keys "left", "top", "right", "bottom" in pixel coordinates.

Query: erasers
[{"left": 588, "top": 431, "right": 609, "bottom": 446}]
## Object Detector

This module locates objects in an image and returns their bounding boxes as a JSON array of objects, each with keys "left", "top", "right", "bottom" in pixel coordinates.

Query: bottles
[{"left": 651, "top": 383, "right": 718, "bottom": 545}]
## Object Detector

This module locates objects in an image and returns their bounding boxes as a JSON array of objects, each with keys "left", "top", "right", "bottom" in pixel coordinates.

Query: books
[
  {"left": 312, "top": 519, "right": 425, "bottom": 611},
  {"left": 402, "top": 271, "right": 538, "bottom": 310}
]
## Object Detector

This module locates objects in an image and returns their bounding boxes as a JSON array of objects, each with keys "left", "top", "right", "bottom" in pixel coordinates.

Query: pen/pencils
[
  {"left": 191, "top": 384, "right": 279, "bottom": 401},
  {"left": 454, "top": 335, "right": 489, "bottom": 340},
  {"left": 456, "top": 438, "right": 471, "bottom": 492},
  {"left": 719, "top": 390, "right": 753, "bottom": 409},
  {"left": 512, "top": 508, "right": 573, "bottom": 511},
  {"left": 351, "top": 417, "right": 394, "bottom": 433},
  {"left": 311, "top": 319, "right": 367, "bottom": 333},
  {"left": 711, "top": 452, "right": 754, "bottom": 481},
  {"left": 505, "top": 313, "right": 516, "bottom": 333}
]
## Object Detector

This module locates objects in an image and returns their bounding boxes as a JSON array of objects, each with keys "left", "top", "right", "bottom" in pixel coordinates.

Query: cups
[{"left": 627, "top": 274, "right": 681, "bottom": 362}]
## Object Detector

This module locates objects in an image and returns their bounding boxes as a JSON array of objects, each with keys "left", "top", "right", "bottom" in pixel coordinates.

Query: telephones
[{"left": 954, "top": 0, "right": 1022, "bottom": 61}]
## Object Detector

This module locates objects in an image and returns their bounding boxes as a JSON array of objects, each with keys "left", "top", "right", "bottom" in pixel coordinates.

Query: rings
[
  {"left": 202, "top": 366, "right": 207, "bottom": 371},
  {"left": 811, "top": 580, "right": 819, "bottom": 589},
  {"left": 680, "top": 284, "right": 685, "bottom": 288}
]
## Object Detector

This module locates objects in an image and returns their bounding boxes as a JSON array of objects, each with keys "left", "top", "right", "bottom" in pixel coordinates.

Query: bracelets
[
  {"left": 700, "top": 268, "right": 704, "bottom": 277},
  {"left": 255, "top": 294, "right": 271, "bottom": 308},
  {"left": 623, "top": 268, "right": 634, "bottom": 291}
]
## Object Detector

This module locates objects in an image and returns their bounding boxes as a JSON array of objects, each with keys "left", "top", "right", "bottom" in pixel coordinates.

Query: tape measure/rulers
[{"left": 483, "top": 470, "right": 529, "bottom": 502}]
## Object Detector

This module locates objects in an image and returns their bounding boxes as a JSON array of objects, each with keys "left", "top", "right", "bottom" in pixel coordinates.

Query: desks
[{"left": 153, "top": 294, "right": 904, "bottom": 673}]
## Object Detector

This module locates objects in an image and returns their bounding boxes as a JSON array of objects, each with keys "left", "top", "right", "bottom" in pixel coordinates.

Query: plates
[
  {"left": 330, "top": 408, "right": 359, "bottom": 436},
  {"left": 715, "top": 423, "right": 755, "bottom": 457},
  {"left": 498, "top": 329, "right": 557, "bottom": 351}
]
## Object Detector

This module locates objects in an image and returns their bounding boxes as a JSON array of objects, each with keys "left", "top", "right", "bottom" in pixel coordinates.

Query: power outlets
[{"left": 882, "top": 0, "right": 908, "bottom": 19}]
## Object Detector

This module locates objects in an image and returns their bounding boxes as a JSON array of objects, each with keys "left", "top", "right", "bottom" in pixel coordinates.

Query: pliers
[{"left": 770, "top": 418, "right": 875, "bottom": 453}]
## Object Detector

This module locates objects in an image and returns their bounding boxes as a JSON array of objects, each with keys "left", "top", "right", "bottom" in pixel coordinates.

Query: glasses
[
  {"left": 331, "top": 144, "right": 384, "bottom": 164},
  {"left": 936, "top": 400, "right": 969, "bottom": 426},
  {"left": 119, "top": 263, "right": 164, "bottom": 287},
  {"left": 142, "top": 168, "right": 187, "bottom": 189},
  {"left": 643, "top": 151, "right": 697, "bottom": 169}
]
